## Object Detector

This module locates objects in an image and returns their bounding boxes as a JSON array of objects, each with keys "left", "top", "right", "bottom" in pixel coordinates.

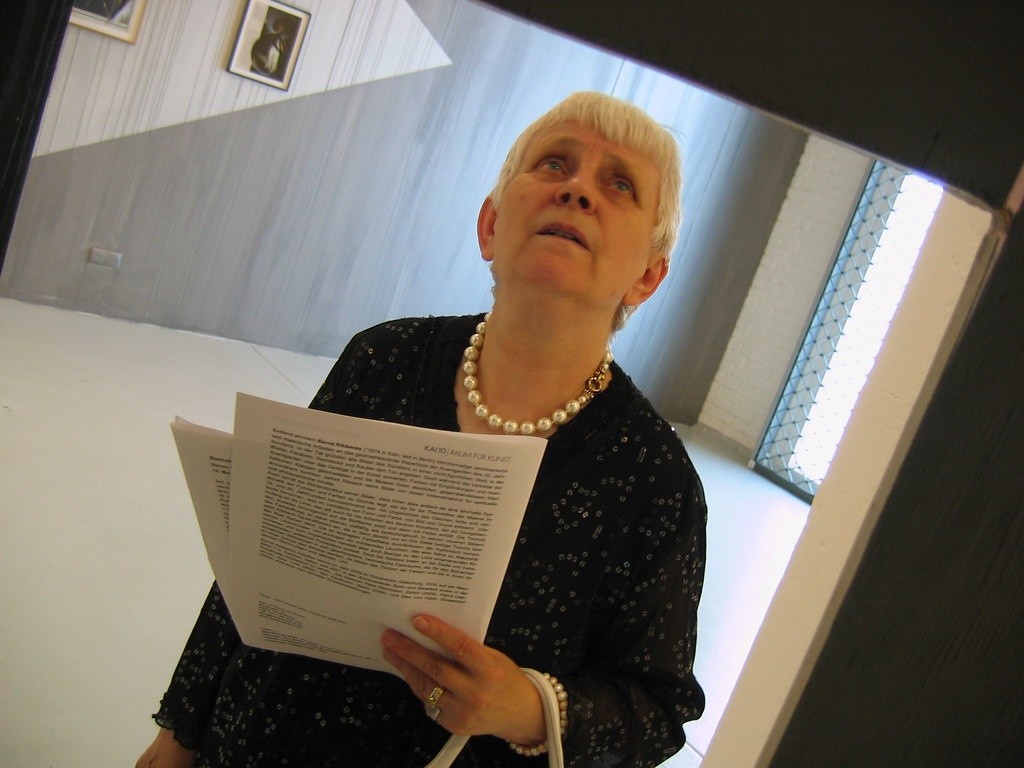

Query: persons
[{"left": 136, "top": 92, "right": 707, "bottom": 768}]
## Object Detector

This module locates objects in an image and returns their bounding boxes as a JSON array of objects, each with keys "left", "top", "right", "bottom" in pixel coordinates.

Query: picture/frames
[
  {"left": 227, "top": 0, "right": 312, "bottom": 90},
  {"left": 71, "top": 0, "right": 149, "bottom": 45}
]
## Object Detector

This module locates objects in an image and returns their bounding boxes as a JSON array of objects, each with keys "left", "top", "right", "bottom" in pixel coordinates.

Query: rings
[
  {"left": 428, "top": 688, "right": 443, "bottom": 706},
  {"left": 425, "top": 704, "right": 440, "bottom": 720}
]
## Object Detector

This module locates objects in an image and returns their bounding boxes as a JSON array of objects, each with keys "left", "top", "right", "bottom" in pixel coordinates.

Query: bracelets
[{"left": 506, "top": 672, "right": 567, "bottom": 756}]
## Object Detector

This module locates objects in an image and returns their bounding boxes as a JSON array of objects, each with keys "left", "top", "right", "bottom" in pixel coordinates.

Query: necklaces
[{"left": 464, "top": 312, "right": 614, "bottom": 434}]
